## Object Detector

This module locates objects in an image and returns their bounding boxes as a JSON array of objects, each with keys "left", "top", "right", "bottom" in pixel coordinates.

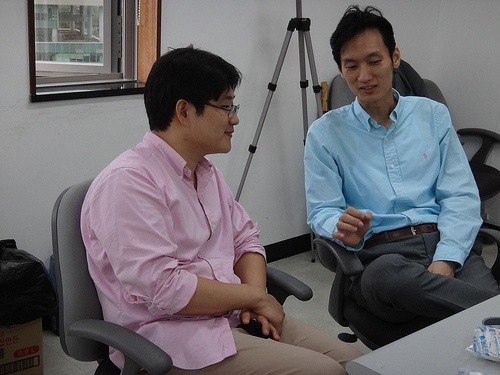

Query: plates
[{"left": 466, "top": 345, "right": 500, "bottom": 363}]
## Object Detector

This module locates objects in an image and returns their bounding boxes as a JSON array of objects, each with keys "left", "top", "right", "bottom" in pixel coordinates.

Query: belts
[{"left": 364, "top": 222, "right": 438, "bottom": 249}]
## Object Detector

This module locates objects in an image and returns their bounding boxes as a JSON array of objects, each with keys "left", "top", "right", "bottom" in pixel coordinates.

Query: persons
[
  {"left": 303, "top": 4, "right": 500, "bottom": 326},
  {"left": 80, "top": 48, "right": 361, "bottom": 375}
]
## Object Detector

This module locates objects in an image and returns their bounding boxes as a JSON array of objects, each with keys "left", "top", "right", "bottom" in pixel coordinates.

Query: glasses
[{"left": 206, "top": 99, "right": 240, "bottom": 119}]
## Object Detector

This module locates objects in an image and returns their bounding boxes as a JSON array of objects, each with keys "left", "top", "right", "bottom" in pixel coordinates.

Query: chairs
[
  {"left": 51, "top": 174, "right": 314, "bottom": 375},
  {"left": 311, "top": 72, "right": 500, "bottom": 352},
  {"left": 420, "top": 78, "right": 500, "bottom": 246}
]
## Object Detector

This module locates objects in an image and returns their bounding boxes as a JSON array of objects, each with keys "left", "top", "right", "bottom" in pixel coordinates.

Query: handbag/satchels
[{"left": 0, "top": 239, "right": 59, "bottom": 326}]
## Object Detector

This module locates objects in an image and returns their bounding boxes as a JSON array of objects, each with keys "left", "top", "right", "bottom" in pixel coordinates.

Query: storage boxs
[{"left": 0, "top": 317, "right": 43, "bottom": 375}]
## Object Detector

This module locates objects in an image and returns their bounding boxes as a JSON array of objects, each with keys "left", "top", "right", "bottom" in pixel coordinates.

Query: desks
[{"left": 344, "top": 293, "right": 500, "bottom": 375}]
